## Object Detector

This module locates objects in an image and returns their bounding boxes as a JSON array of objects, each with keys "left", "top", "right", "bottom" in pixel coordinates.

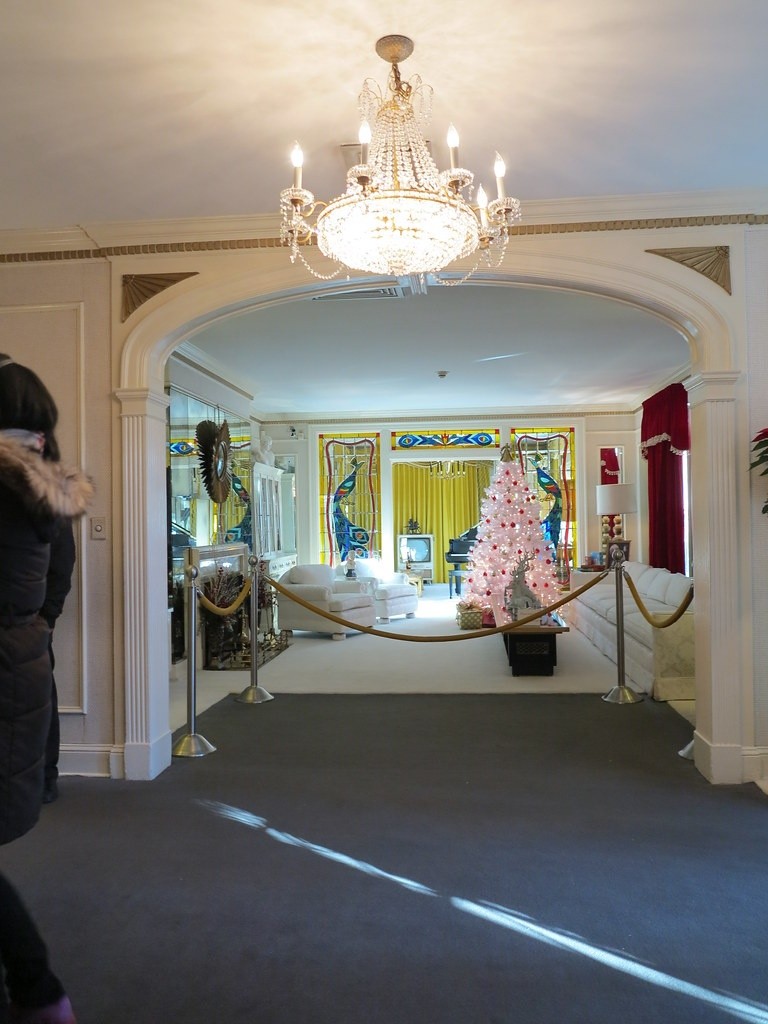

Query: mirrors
[{"left": 167, "top": 387, "right": 252, "bottom": 588}]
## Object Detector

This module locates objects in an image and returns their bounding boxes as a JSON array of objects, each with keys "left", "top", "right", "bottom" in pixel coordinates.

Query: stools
[
  {"left": 448, "top": 570, "right": 474, "bottom": 599},
  {"left": 402, "top": 569, "right": 424, "bottom": 597}
]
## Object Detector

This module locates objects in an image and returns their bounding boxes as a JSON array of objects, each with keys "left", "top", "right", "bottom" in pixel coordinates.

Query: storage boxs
[{"left": 455, "top": 602, "right": 483, "bottom": 630}]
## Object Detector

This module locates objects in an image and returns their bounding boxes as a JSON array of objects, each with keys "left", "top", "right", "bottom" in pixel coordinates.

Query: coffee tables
[{"left": 491, "top": 600, "right": 570, "bottom": 676}]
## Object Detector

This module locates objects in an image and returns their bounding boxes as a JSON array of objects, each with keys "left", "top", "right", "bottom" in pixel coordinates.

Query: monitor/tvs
[{"left": 397, "top": 535, "right": 432, "bottom": 570}]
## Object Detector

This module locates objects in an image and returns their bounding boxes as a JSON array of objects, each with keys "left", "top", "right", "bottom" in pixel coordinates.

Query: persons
[
  {"left": 344, "top": 550, "right": 356, "bottom": 574},
  {"left": 255, "top": 436, "right": 276, "bottom": 467},
  {"left": 0, "top": 355, "right": 98, "bottom": 1022}
]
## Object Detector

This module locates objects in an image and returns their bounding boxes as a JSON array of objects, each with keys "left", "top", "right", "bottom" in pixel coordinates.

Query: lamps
[
  {"left": 278, "top": 35, "right": 522, "bottom": 286},
  {"left": 429, "top": 460, "right": 466, "bottom": 480},
  {"left": 596, "top": 483, "right": 639, "bottom": 571}
]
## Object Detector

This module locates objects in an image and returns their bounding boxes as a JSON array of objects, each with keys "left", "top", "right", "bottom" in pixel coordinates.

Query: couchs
[
  {"left": 568, "top": 560, "right": 696, "bottom": 701},
  {"left": 276, "top": 563, "right": 377, "bottom": 640},
  {"left": 335, "top": 558, "right": 419, "bottom": 624}
]
